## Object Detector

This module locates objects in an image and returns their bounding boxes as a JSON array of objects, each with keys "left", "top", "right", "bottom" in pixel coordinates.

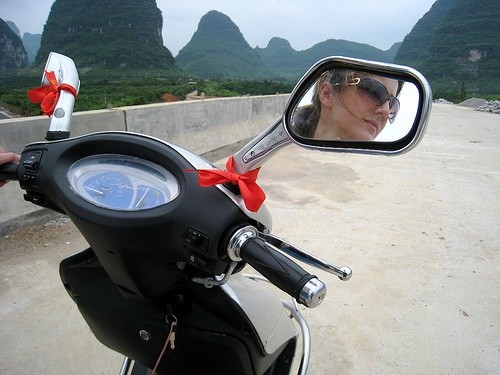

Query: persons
[
  {"left": 0, "top": 148, "right": 22, "bottom": 188},
  {"left": 290, "top": 69, "right": 403, "bottom": 142}
]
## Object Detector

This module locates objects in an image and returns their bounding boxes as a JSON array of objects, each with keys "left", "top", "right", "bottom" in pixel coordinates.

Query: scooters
[{"left": 0, "top": 51, "right": 431, "bottom": 375}]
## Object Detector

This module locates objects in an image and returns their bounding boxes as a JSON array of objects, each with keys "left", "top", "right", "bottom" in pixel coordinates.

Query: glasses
[{"left": 333, "top": 77, "right": 401, "bottom": 124}]
